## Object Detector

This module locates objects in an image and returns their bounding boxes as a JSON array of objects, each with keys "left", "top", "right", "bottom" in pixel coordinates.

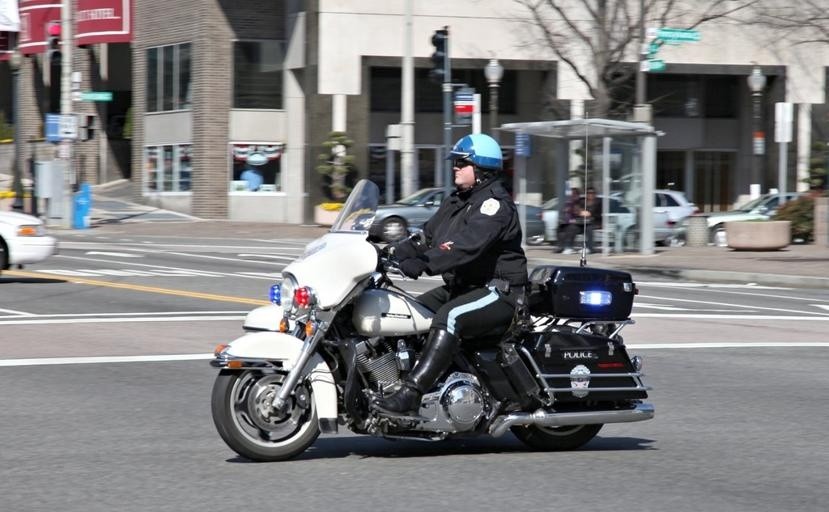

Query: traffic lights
[
  {"left": 39, "top": 21, "right": 62, "bottom": 87},
  {"left": 431, "top": 30, "right": 447, "bottom": 84}
]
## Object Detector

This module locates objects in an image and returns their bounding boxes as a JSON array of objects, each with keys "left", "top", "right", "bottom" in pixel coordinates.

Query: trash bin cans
[
  {"left": 73, "top": 184, "right": 92, "bottom": 231},
  {"left": 687, "top": 214, "right": 709, "bottom": 247}
]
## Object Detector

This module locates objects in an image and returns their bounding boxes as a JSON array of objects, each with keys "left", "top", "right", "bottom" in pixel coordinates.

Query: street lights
[
  {"left": 749, "top": 69, "right": 765, "bottom": 196},
  {"left": 483, "top": 60, "right": 505, "bottom": 142},
  {"left": 10, "top": 50, "right": 29, "bottom": 206}
]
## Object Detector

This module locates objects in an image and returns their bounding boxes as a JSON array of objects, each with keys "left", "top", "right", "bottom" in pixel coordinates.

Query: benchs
[{"left": 542, "top": 210, "right": 636, "bottom": 254}]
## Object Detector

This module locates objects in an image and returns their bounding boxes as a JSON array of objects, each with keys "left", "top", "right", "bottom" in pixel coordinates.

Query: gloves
[{"left": 394, "top": 240, "right": 428, "bottom": 280}]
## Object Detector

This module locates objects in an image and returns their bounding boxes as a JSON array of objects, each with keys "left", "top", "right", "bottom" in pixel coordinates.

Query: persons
[
  {"left": 561, "top": 186, "right": 604, "bottom": 253},
  {"left": 554, "top": 188, "right": 579, "bottom": 253},
  {"left": 373, "top": 132, "right": 540, "bottom": 417}
]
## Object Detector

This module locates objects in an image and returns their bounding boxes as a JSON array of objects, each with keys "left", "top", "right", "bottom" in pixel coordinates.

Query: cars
[
  {"left": 615, "top": 187, "right": 700, "bottom": 242},
  {"left": 673, "top": 191, "right": 812, "bottom": 250},
  {"left": 353, "top": 189, "right": 545, "bottom": 242},
  {"left": 539, "top": 195, "right": 640, "bottom": 253},
  {"left": 1, "top": 211, "right": 57, "bottom": 266}
]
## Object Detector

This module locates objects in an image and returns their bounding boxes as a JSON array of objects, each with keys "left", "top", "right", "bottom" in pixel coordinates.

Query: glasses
[{"left": 453, "top": 157, "right": 474, "bottom": 169}]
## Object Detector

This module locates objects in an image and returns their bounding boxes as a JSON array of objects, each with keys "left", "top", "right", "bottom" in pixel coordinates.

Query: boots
[{"left": 371, "top": 327, "right": 461, "bottom": 416}]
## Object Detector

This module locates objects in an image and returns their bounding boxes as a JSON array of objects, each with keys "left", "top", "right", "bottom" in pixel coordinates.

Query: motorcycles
[{"left": 209, "top": 178, "right": 653, "bottom": 463}]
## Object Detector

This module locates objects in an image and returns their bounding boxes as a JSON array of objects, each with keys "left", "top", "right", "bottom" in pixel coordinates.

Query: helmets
[{"left": 443, "top": 134, "right": 504, "bottom": 170}]
